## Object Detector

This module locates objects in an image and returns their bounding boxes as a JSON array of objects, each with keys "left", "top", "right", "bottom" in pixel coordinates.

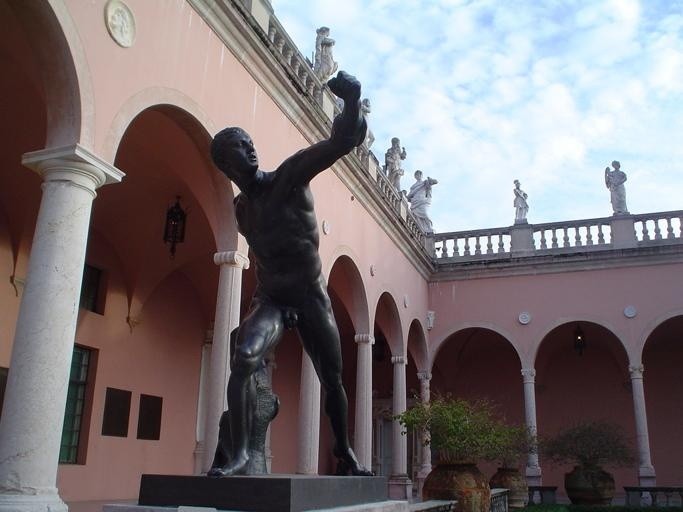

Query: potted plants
[{"left": 384, "top": 388, "right": 643, "bottom": 512}]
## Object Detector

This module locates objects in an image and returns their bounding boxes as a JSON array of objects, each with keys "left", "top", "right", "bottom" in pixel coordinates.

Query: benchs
[{"left": 621, "top": 484, "right": 683, "bottom": 511}]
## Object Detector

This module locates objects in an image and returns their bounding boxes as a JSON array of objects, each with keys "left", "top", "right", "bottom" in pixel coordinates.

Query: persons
[
  {"left": 402, "top": 171, "right": 438, "bottom": 233},
  {"left": 314, "top": 27, "right": 338, "bottom": 82},
  {"left": 514, "top": 180, "right": 529, "bottom": 220},
  {"left": 210, "top": 70, "right": 373, "bottom": 475},
  {"left": 386, "top": 138, "right": 407, "bottom": 195},
  {"left": 605, "top": 161, "right": 627, "bottom": 213},
  {"left": 361, "top": 99, "right": 375, "bottom": 148}
]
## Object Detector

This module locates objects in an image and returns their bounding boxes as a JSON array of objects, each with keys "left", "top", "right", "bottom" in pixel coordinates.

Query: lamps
[{"left": 161, "top": 193, "right": 186, "bottom": 258}]
